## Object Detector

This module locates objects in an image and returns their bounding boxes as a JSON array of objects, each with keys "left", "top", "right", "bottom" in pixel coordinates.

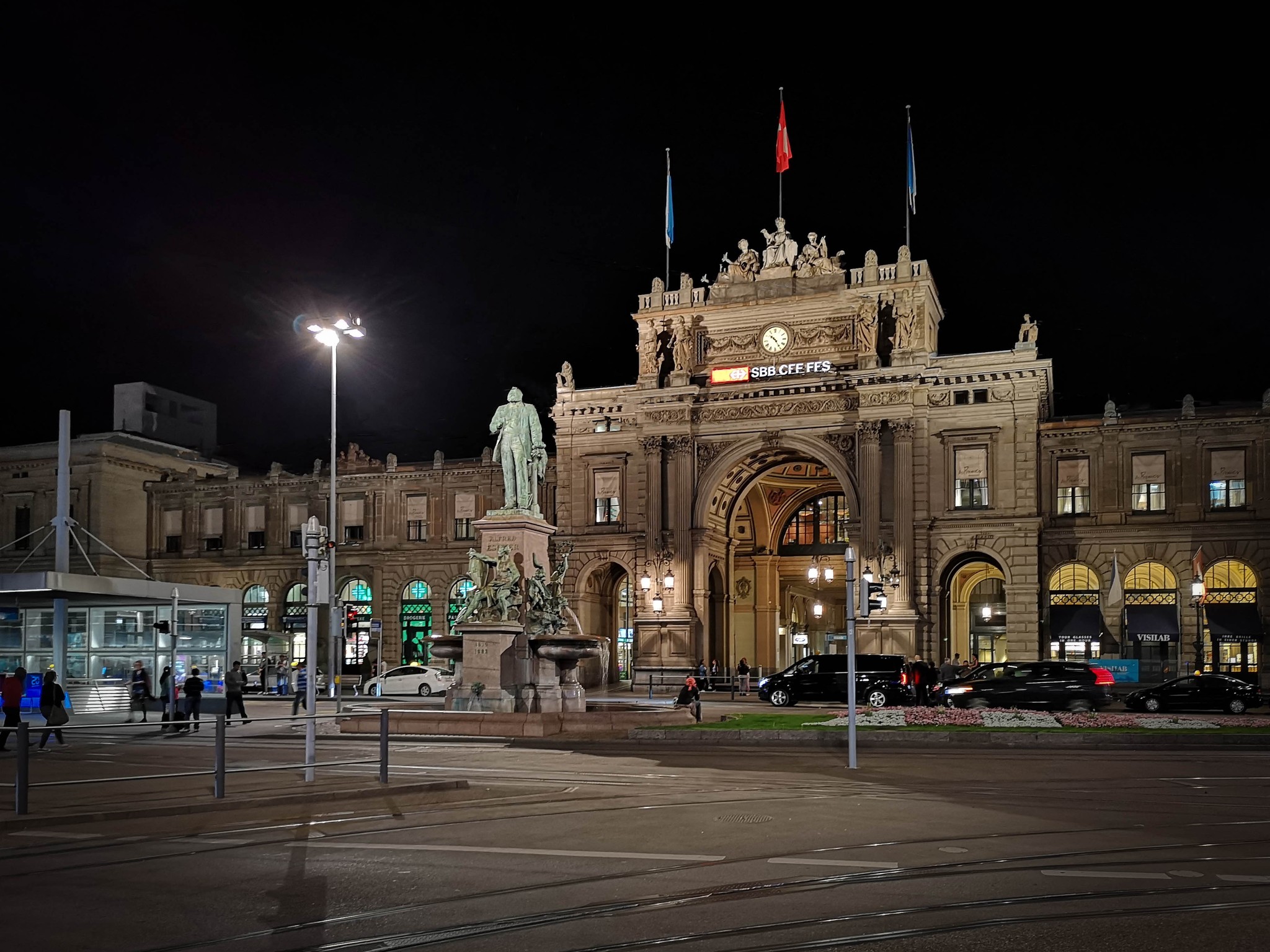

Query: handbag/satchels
[{"left": 49, "top": 704, "right": 69, "bottom": 725}]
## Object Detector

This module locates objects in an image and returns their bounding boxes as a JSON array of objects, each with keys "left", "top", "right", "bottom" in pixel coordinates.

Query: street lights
[
  {"left": 1188, "top": 570, "right": 1205, "bottom": 672},
  {"left": 303, "top": 312, "right": 368, "bottom": 695}
]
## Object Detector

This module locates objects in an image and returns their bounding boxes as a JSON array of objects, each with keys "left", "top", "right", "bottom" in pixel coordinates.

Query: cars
[
  {"left": 362, "top": 665, "right": 455, "bottom": 697},
  {"left": 925, "top": 660, "right": 1116, "bottom": 713},
  {"left": 242, "top": 664, "right": 327, "bottom": 694},
  {"left": 1124, "top": 670, "right": 1265, "bottom": 715}
]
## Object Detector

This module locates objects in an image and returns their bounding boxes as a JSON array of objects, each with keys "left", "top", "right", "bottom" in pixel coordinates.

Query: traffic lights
[{"left": 345, "top": 604, "right": 359, "bottom": 640}]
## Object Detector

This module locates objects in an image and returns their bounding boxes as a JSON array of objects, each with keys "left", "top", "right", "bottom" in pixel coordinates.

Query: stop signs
[{"left": 859, "top": 580, "right": 883, "bottom": 617}]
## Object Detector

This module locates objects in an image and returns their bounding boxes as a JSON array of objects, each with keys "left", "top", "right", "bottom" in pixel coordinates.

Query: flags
[
  {"left": 908, "top": 117, "right": 917, "bottom": 214},
  {"left": 1192, "top": 550, "right": 1206, "bottom": 606},
  {"left": 775, "top": 100, "right": 792, "bottom": 173},
  {"left": 666, "top": 175, "right": 674, "bottom": 249},
  {"left": 1107, "top": 554, "right": 1122, "bottom": 607}
]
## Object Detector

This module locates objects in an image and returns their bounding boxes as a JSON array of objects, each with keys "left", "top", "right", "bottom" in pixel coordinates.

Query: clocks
[{"left": 761, "top": 325, "right": 789, "bottom": 354}]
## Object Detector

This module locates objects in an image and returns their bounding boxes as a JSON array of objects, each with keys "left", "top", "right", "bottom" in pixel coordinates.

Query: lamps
[
  {"left": 982, "top": 564, "right": 992, "bottom": 622},
  {"left": 807, "top": 555, "right": 835, "bottom": 590},
  {"left": 813, "top": 599, "right": 824, "bottom": 619},
  {"left": 862, "top": 541, "right": 902, "bottom": 613},
  {"left": 640, "top": 537, "right": 676, "bottom": 616}
]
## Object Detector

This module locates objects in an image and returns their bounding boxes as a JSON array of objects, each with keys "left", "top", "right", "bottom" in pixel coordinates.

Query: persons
[
  {"left": 909, "top": 653, "right": 978, "bottom": 707},
  {"left": 122, "top": 650, "right": 308, "bottom": 733},
  {"left": 1019, "top": 314, "right": 1038, "bottom": 342},
  {"left": 340, "top": 442, "right": 382, "bottom": 467},
  {"left": 676, "top": 678, "right": 702, "bottom": 724},
  {"left": 699, "top": 657, "right": 751, "bottom": 696},
  {"left": 855, "top": 289, "right": 913, "bottom": 353},
  {"left": 488, "top": 386, "right": 546, "bottom": 511},
  {"left": 815, "top": 650, "right": 822, "bottom": 655},
  {"left": 641, "top": 315, "right": 695, "bottom": 373},
  {"left": 701, "top": 219, "right": 846, "bottom": 286},
  {"left": 1104, "top": 388, "right": 1270, "bottom": 418},
  {"left": 556, "top": 361, "right": 573, "bottom": 387},
  {"left": 0, "top": 666, "right": 72, "bottom": 753},
  {"left": 455, "top": 538, "right": 583, "bottom": 637},
  {"left": 369, "top": 656, "right": 388, "bottom": 679}
]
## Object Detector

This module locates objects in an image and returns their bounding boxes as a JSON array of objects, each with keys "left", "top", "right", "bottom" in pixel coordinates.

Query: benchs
[{"left": 315, "top": 674, "right": 362, "bottom": 696}]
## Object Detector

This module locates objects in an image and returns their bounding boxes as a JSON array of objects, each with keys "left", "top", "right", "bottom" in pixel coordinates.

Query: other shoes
[
  {"left": 37, "top": 748, "right": 52, "bottom": 752},
  {"left": 226, "top": 722, "right": 231, "bottom": 725},
  {"left": 124, "top": 719, "right": 134, "bottom": 722},
  {"left": 138, "top": 719, "right": 147, "bottom": 722},
  {"left": 179, "top": 728, "right": 190, "bottom": 732},
  {"left": 242, "top": 720, "right": 252, "bottom": 724},
  {"left": 740, "top": 693, "right": 746, "bottom": 696},
  {"left": 161, "top": 728, "right": 167, "bottom": 732},
  {"left": 194, "top": 729, "right": 198, "bottom": 732},
  {"left": 59, "top": 743, "right": 67, "bottom": 747},
  {"left": 1, "top": 748, "right": 11, "bottom": 752}
]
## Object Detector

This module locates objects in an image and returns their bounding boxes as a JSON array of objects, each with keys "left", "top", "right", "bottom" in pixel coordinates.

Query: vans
[{"left": 758, "top": 653, "right": 916, "bottom": 708}]
